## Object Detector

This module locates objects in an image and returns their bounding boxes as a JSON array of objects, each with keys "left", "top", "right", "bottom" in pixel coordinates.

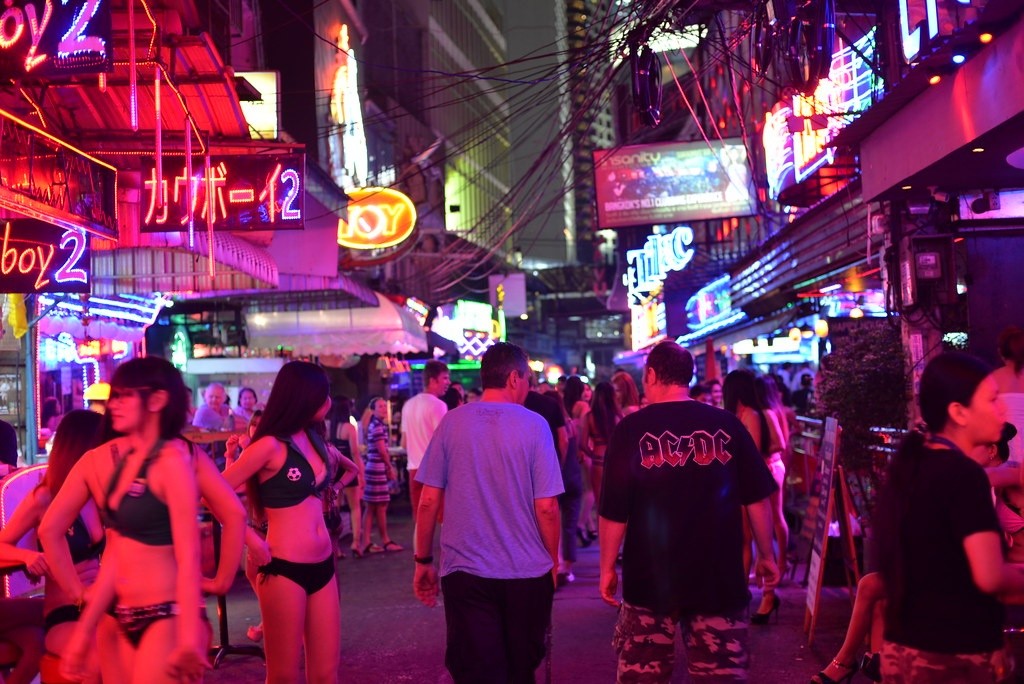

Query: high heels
[
  {"left": 750, "top": 595, "right": 780, "bottom": 625},
  {"left": 335, "top": 550, "right": 346, "bottom": 560},
  {"left": 776, "top": 561, "right": 792, "bottom": 585},
  {"left": 351, "top": 543, "right": 371, "bottom": 559},
  {"left": 747, "top": 564, "right": 760, "bottom": 585},
  {"left": 575, "top": 523, "right": 598, "bottom": 548},
  {"left": 811, "top": 658, "right": 859, "bottom": 684}
]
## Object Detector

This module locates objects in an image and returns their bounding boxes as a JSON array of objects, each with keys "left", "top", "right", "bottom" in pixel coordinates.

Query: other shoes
[
  {"left": 557, "top": 564, "right": 574, "bottom": 585},
  {"left": 363, "top": 541, "right": 384, "bottom": 554},
  {"left": 384, "top": 541, "right": 403, "bottom": 551}
]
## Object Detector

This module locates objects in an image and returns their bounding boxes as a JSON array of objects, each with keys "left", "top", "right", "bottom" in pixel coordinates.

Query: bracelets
[{"left": 414, "top": 554, "right": 433, "bottom": 564}]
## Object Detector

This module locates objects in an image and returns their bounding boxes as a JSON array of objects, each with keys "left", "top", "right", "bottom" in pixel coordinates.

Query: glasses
[{"left": 109, "top": 386, "right": 154, "bottom": 405}]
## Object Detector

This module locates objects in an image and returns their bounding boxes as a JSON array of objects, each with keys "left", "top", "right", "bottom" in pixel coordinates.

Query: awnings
[{"left": 248, "top": 291, "right": 427, "bottom": 355}]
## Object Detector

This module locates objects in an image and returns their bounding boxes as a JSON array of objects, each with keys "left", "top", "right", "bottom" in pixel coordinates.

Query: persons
[
  {"left": 184, "top": 383, "right": 257, "bottom": 471},
  {"left": 0, "top": 355, "right": 247, "bottom": 684},
  {"left": 413, "top": 343, "right": 565, "bottom": 684},
  {"left": 203, "top": 362, "right": 340, "bottom": 684},
  {"left": 402, "top": 362, "right": 480, "bottom": 527},
  {"left": 526, "top": 367, "right": 648, "bottom": 584},
  {"left": 877, "top": 356, "right": 1024, "bottom": 684},
  {"left": 810, "top": 422, "right": 1024, "bottom": 684},
  {"left": 600, "top": 341, "right": 781, "bottom": 684},
  {"left": 324, "top": 397, "right": 405, "bottom": 557},
  {"left": 691, "top": 337, "right": 813, "bottom": 623}
]
